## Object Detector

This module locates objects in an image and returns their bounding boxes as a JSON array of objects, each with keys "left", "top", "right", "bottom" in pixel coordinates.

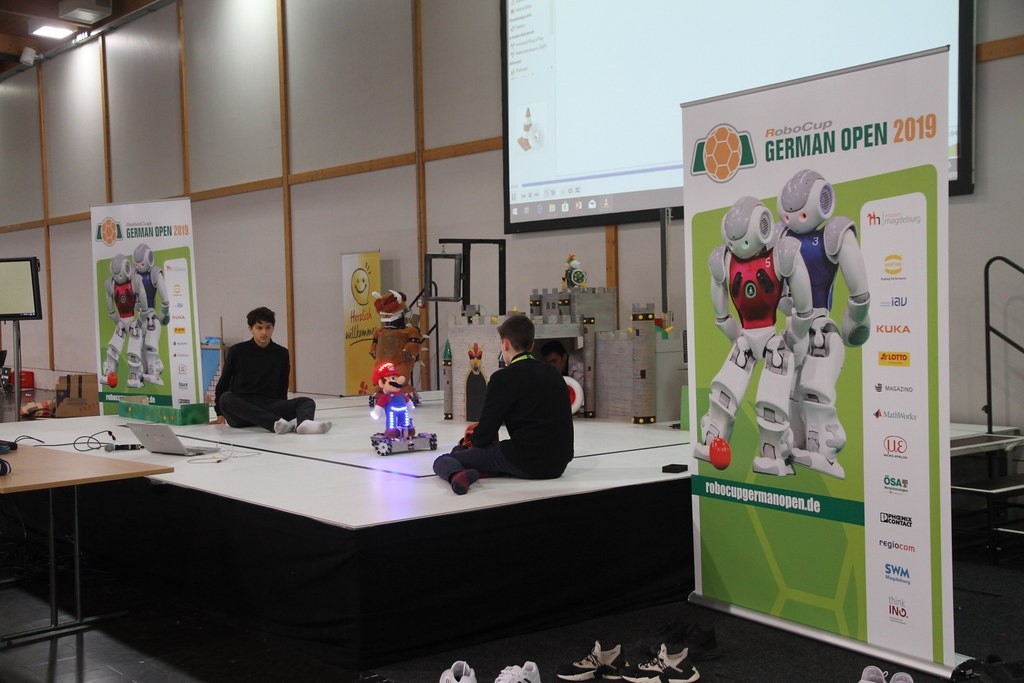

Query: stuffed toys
[{"left": 368, "top": 290, "right": 422, "bottom": 399}]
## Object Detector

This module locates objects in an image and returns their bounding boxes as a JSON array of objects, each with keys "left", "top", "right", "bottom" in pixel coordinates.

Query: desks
[{"left": 0, "top": 438, "right": 174, "bottom": 641}]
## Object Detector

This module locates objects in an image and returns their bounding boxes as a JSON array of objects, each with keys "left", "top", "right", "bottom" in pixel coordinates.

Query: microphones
[{"left": 105, "top": 444, "right": 145, "bottom": 452}]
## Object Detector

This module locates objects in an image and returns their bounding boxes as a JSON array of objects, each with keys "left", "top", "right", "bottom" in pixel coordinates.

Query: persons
[
  {"left": 371, "top": 360, "right": 416, "bottom": 438},
  {"left": 209, "top": 307, "right": 332, "bottom": 434},
  {"left": 435, "top": 315, "right": 573, "bottom": 493},
  {"left": 542, "top": 341, "right": 584, "bottom": 388}
]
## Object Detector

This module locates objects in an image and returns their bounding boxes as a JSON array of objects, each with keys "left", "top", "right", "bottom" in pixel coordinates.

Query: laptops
[{"left": 127, "top": 423, "right": 220, "bottom": 456}]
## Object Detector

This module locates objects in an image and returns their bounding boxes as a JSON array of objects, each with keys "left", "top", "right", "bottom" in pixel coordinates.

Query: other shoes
[
  {"left": 985, "top": 653, "right": 1023, "bottom": 683},
  {"left": 858, "top": 666, "right": 888, "bottom": 683},
  {"left": 890, "top": 672, "right": 914, "bottom": 683}
]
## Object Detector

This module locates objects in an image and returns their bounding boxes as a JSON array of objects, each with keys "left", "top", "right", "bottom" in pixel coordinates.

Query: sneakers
[
  {"left": 619, "top": 643, "right": 700, "bottom": 683},
  {"left": 556, "top": 640, "right": 623, "bottom": 681},
  {"left": 439, "top": 661, "right": 477, "bottom": 683},
  {"left": 494, "top": 660, "right": 541, "bottom": 683}
]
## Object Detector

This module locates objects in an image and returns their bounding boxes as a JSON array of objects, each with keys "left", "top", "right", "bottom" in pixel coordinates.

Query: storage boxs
[
  {"left": 55, "top": 374, "right": 100, "bottom": 418},
  {"left": 9, "top": 369, "right": 34, "bottom": 391}
]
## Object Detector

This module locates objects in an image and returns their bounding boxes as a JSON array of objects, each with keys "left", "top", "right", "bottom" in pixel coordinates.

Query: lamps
[
  {"left": 28, "top": 19, "right": 78, "bottom": 43},
  {"left": 58, "top": 0, "right": 113, "bottom": 25},
  {"left": 20, "top": 47, "right": 44, "bottom": 66}
]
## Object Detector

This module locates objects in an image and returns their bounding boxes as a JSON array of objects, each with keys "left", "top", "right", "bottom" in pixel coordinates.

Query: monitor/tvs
[{"left": 0, "top": 256, "right": 42, "bottom": 321}]
